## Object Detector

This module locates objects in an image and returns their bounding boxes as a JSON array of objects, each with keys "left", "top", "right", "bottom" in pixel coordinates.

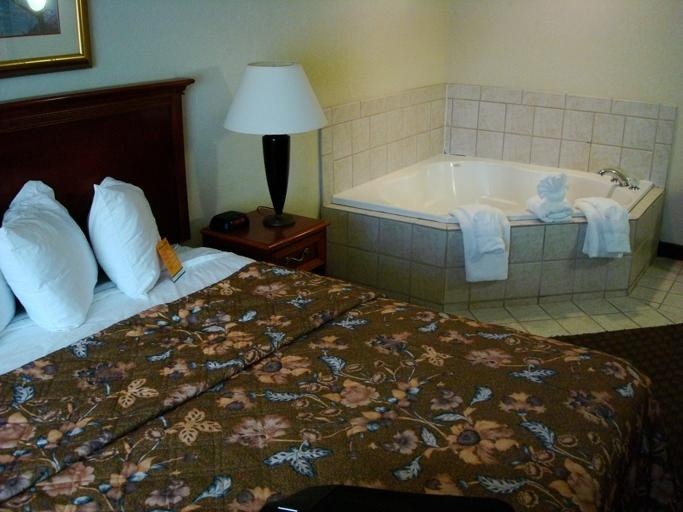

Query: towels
[{"left": 450, "top": 173, "right": 631, "bottom": 283}]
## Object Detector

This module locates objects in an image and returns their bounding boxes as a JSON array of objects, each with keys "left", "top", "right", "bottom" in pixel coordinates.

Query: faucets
[{"left": 597, "top": 167, "right": 629, "bottom": 187}]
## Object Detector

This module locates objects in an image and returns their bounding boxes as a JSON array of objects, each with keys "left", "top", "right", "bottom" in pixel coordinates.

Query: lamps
[{"left": 223, "top": 61, "right": 328, "bottom": 228}]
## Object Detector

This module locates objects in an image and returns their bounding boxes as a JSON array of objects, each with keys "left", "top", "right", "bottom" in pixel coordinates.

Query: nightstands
[{"left": 200, "top": 209, "right": 331, "bottom": 274}]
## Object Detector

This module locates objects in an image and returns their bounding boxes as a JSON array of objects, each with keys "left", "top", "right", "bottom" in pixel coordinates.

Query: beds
[{"left": 0, "top": 78, "right": 683, "bottom": 512}]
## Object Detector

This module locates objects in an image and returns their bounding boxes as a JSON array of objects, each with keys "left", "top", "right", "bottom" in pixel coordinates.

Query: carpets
[{"left": 546, "top": 323, "right": 683, "bottom": 496}]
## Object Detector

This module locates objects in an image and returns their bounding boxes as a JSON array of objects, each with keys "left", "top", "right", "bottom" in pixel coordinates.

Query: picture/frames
[{"left": 0, "top": 0, "right": 95, "bottom": 77}]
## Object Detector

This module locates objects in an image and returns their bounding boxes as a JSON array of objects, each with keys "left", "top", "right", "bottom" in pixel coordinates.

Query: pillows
[{"left": 0, "top": 176, "right": 166, "bottom": 333}]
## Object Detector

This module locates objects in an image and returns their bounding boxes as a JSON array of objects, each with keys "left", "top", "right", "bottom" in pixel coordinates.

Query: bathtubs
[{"left": 330, "top": 153, "right": 656, "bottom": 224}]
